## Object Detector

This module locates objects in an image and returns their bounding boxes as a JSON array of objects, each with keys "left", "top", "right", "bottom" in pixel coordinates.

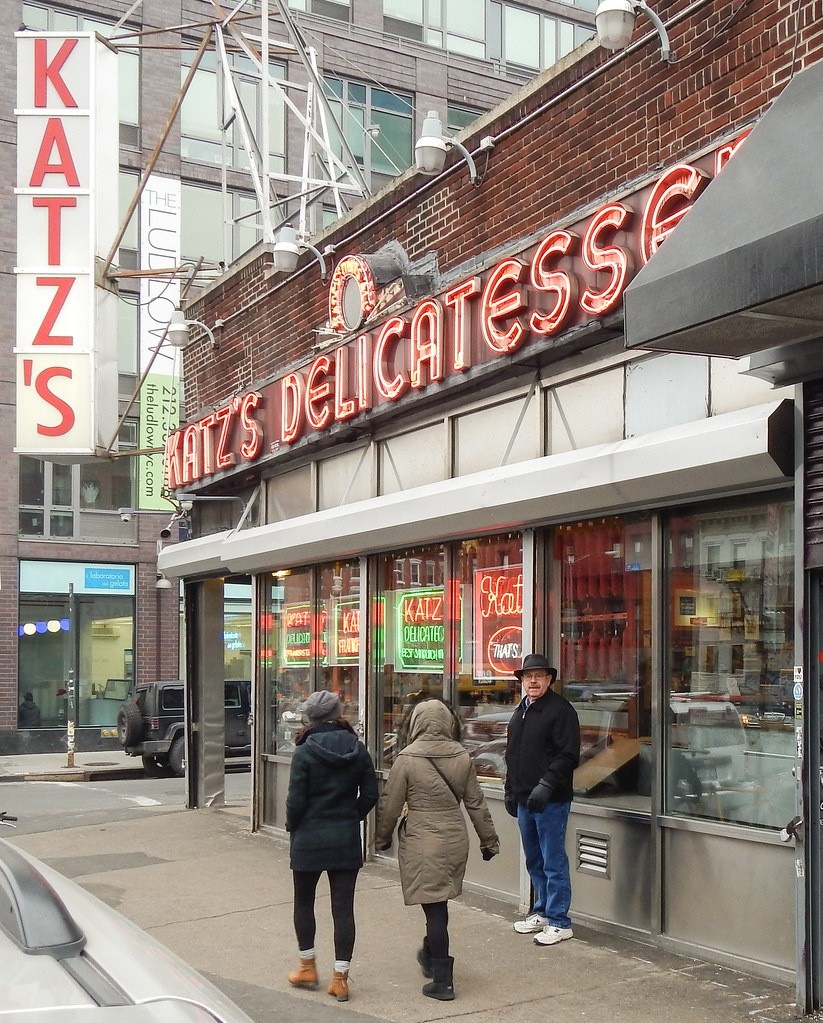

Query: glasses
[{"left": 522, "top": 673, "right": 551, "bottom": 682}]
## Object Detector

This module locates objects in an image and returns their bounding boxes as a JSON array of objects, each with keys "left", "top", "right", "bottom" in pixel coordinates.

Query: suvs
[
  {"left": 117, "top": 680, "right": 253, "bottom": 778},
  {"left": 460, "top": 678, "right": 796, "bottom": 810}
]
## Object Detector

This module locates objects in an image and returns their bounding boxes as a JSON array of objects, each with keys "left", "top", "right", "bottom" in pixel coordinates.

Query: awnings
[{"left": 624, "top": 58, "right": 823, "bottom": 390}]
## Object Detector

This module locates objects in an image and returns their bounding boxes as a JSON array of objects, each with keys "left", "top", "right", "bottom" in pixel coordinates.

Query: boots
[
  {"left": 288, "top": 958, "right": 318, "bottom": 987},
  {"left": 422, "top": 956, "right": 455, "bottom": 1001},
  {"left": 327, "top": 968, "right": 353, "bottom": 1002},
  {"left": 417, "top": 935, "right": 433, "bottom": 978}
]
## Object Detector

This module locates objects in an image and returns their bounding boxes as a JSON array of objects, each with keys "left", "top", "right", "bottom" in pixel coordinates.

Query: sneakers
[
  {"left": 513, "top": 914, "right": 549, "bottom": 934},
  {"left": 533, "top": 926, "right": 573, "bottom": 945}
]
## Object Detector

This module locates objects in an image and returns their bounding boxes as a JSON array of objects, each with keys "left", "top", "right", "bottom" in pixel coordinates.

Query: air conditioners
[{"left": 91, "top": 626, "right": 120, "bottom": 639}]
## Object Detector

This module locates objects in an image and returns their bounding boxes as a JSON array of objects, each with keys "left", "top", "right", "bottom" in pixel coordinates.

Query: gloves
[
  {"left": 527, "top": 778, "right": 557, "bottom": 815},
  {"left": 505, "top": 790, "right": 518, "bottom": 818}
]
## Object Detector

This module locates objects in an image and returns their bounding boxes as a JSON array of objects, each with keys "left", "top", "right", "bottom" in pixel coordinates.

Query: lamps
[
  {"left": 414, "top": 109, "right": 481, "bottom": 185},
  {"left": 167, "top": 309, "right": 219, "bottom": 351},
  {"left": 176, "top": 493, "right": 252, "bottom": 522},
  {"left": 594, "top": 0, "right": 670, "bottom": 63},
  {"left": 154, "top": 562, "right": 173, "bottom": 590},
  {"left": 272, "top": 221, "right": 328, "bottom": 282}
]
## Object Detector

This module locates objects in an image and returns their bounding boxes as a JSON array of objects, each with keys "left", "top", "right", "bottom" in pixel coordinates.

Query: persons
[
  {"left": 393, "top": 691, "right": 402, "bottom": 704},
  {"left": 286, "top": 690, "right": 380, "bottom": 1002},
  {"left": 373, "top": 688, "right": 501, "bottom": 1002},
  {"left": 605, "top": 657, "right": 704, "bottom": 800},
  {"left": 18, "top": 692, "right": 42, "bottom": 728},
  {"left": 504, "top": 653, "right": 582, "bottom": 946}
]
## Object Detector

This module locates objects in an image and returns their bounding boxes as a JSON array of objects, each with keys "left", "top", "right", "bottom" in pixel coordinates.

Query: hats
[
  {"left": 302, "top": 690, "right": 342, "bottom": 723},
  {"left": 514, "top": 654, "right": 557, "bottom": 687}
]
